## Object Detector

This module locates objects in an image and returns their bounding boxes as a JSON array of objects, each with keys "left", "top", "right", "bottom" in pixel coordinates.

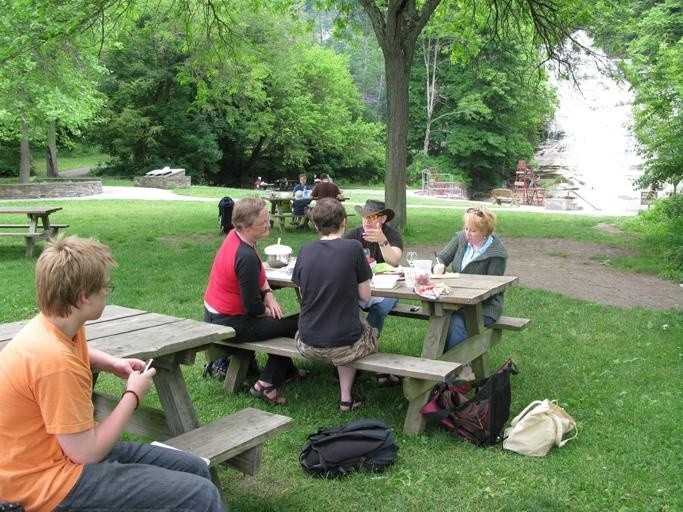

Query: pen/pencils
[{"left": 434, "top": 250, "right": 440, "bottom": 265}]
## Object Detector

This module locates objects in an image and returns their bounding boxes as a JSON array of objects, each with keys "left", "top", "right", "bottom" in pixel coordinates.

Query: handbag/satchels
[
  {"left": 503, "top": 398, "right": 578, "bottom": 457},
  {"left": 202, "top": 357, "right": 261, "bottom": 380},
  {"left": 420, "top": 358, "right": 518, "bottom": 445}
]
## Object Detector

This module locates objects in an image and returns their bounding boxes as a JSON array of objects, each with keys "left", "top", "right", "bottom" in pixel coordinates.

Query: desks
[
  {"left": 0, "top": 304, "right": 238, "bottom": 511},
  {"left": 264, "top": 196, "right": 351, "bottom": 228},
  {"left": 260, "top": 183, "right": 275, "bottom": 191},
  {"left": 222, "top": 257, "right": 518, "bottom": 435},
  {"left": 1, "top": 207, "right": 62, "bottom": 256}
]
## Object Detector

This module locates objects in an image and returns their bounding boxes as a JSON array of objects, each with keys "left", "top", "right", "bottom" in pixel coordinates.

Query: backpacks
[{"left": 299, "top": 420, "right": 400, "bottom": 479}]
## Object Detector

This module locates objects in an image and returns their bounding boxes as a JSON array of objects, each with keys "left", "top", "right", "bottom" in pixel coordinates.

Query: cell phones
[
  {"left": 410, "top": 306, "right": 420, "bottom": 312},
  {"left": 140, "top": 358, "right": 153, "bottom": 375}
]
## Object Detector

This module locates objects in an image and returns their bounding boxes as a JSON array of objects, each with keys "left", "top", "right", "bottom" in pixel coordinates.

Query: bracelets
[
  {"left": 122, "top": 390, "right": 141, "bottom": 414},
  {"left": 379, "top": 239, "right": 389, "bottom": 248}
]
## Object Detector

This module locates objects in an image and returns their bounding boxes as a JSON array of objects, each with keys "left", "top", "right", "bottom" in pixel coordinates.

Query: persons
[
  {"left": 430, "top": 206, "right": 509, "bottom": 384},
  {"left": 1, "top": 231, "right": 229, "bottom": 512},
  {"left": 255, "top": 176, "right": 288, "bottom": 191},
  {"left": 291, "top": 198, "right": 401, "bottom": 412},
  {"left": 204, "top": 197, "right": 298, "bottom": 405},
  {"left": 343, "top": 199, "right": 403, "bottom": 340},
  {"left": 289, "top": 172, "right": 345, "bottom": 232}
]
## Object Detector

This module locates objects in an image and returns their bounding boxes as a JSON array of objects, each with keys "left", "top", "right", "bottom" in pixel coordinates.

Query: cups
[{"left": 403, "top": 268, "right": 417, "bottom": 288}]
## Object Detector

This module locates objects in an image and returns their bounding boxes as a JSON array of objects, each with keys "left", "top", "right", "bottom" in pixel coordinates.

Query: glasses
[
  {"left": 100, "top": 281, "right": 114, "bottom": 292},
  {"left": 363, "top": 215, "right": 379, "bottom": 221}
]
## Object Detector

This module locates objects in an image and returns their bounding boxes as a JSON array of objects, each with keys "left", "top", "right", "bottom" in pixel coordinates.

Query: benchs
[
  {"left": 490, "top": 187, "right": 519, "bottom": 207},
  {"left": 154, "top": 406, "right": 294, "bottom": 470},
  {"left": 275, "top": 188, "right": 280, "bottom": 191},
  {"left": 390, "top": 303, "right": 532, "bottom": 331},
  {"left": 274, "top": 213, "right": 356, "bottom": 218},
  {"left": 0, "top": 231, "right": 44, "bottom": 238},
  {"left": 213, "top": 335, "right": 461, "bottom": 383},
  {"left": 0, "top": 223, "right": 70, "bottom": 228}
]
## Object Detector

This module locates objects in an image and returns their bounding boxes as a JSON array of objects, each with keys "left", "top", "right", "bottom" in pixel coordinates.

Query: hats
[
  {"left": 295, "top": 190, "right": 304, "bottom": 201},
  {"left": 355, "top": 199, "right": 395, "bottom": 223}
]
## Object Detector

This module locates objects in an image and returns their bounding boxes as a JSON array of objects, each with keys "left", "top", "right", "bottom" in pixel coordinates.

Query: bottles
[{"left": 304, "top": 189, "right": 307, "bottom": 197}]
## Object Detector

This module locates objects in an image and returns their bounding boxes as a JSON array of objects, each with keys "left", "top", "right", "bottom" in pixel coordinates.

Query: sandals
[
  {"left": 249, "top": 380, "right": 287, "bottom": 405},
  {"left": 376, "top": 373, "right": 399, "bottom": 388},
  {"left": 337, "top": 395, "right": 365, "bottom": 412},
  {"left": 300, "top": 368, "right": 311, "bottom": 381}
]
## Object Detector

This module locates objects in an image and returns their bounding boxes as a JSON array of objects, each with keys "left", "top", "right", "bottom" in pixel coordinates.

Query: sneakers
[{"left": 453, "top": 365, "right": 475, "bottom": 382}]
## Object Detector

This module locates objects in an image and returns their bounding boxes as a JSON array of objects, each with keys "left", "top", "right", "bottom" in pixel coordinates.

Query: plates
[{"left": 264, "top": 244, "right": 292, "bottom": 255}]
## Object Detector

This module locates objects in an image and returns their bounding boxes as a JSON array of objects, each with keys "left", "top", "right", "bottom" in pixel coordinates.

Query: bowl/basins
[{"left": 265, "top": 254, "right": 290, "bottom": 268}]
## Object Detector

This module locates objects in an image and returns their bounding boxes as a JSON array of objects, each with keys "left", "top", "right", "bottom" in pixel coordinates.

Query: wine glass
[
  {"left": 363, "top": 248, "right": 370, "bottom": 260},
  {"left": 406, "top": 252, "right": 416, "bottom": 273}
]
